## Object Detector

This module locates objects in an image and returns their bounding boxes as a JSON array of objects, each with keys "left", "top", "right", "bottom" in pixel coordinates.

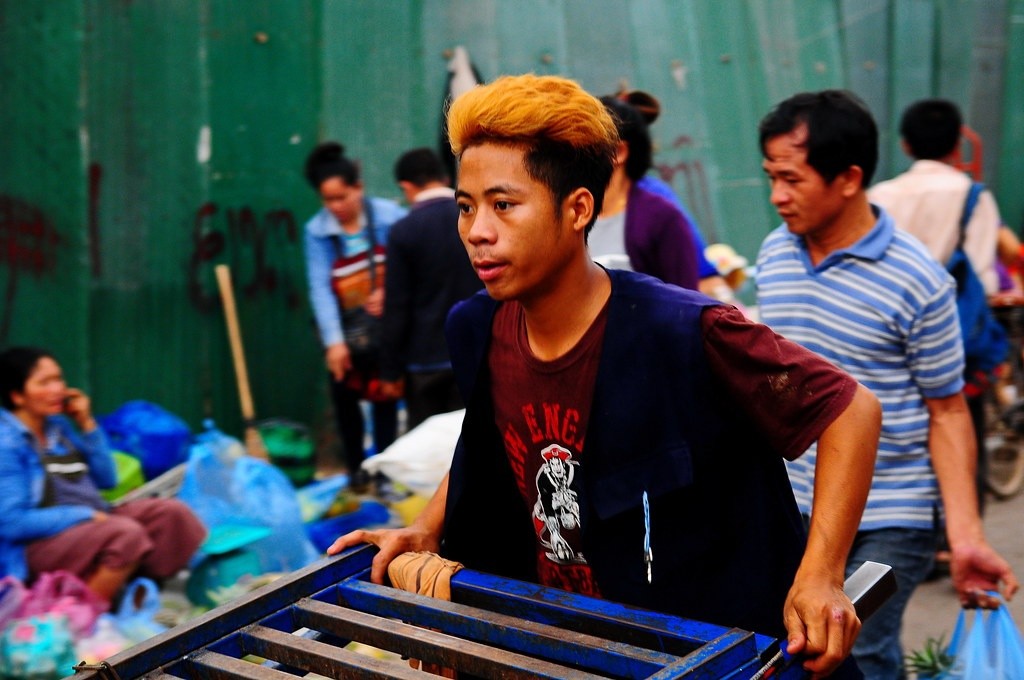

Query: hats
[{"left": 702, "top": 243, "right": 748, "bottom": 276}]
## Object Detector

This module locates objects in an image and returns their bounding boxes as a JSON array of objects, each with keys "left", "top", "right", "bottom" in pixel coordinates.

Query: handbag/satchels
[
  {"left": 329, "top": 322, "right": 405, "bottom": 383},
  {"left": 172, "top": 442, "right": 320, "bottom": 590},
  {"left": 928, "top": 591, "right": 1024, "bottom": 680},
  {"left": 941, "top": 183, "right": 1007, "bottom": 371},
  {"left": 90, "top": 579, "right": 168, "bottom": 645}
]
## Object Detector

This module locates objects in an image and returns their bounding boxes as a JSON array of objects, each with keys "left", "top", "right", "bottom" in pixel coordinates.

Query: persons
[
  {"left": 755, "top": 88, "right": 1020, "bottom": 680},
  {"left": 300, "top": 72, "right": 1024, "bottom": 680},
  {"left": 0, "top": 346, "right": 207, "bottom": 615},
  {"left": 326, "top": 74, "right": 883, "bottom": 680}
]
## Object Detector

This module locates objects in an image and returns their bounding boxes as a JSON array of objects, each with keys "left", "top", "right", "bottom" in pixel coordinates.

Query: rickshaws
[{"left": 54, "top": 537, "right": 899, "bottom": 680}]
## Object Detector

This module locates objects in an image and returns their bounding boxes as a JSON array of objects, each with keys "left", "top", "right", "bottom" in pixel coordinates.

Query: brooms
[{"left": 214, "top": 263, "right": 270, "bottom": 461}]
